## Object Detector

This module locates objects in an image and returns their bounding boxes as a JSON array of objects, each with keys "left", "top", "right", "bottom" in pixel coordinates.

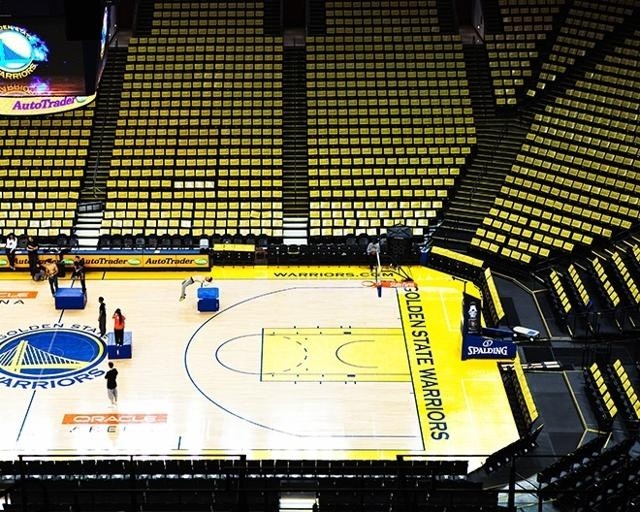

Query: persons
[
  {"left": 178, "top": 274, "right": 213, "bottom": 302},
  {"left": 97, "top": 296, "right": 107, "bottom": 338},
  {"left": 5, "top": 233, "right": 18, "bottom": 269},
  {"left": 104, "top": 362, "right": 119, "bottom": 408},
  {"left": 25, "top": 236, "right": 88, "bottom": 296},
  {"left": 366, "top": 239, "right": 381, "bottom": 273},
  {"left": 111, "top": 309, "right": 126, "bottom": 345}
]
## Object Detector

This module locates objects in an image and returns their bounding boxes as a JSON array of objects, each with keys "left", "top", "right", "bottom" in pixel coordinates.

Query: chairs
[
  {"left": 536, "top": 246, "right": 640, "bottom": 512},
  {"left": 0, "top": 0, "right": 640, "bottom": 269},
  {"left": 0, "top": 458, "right": 500, "bottom": 512}
]
